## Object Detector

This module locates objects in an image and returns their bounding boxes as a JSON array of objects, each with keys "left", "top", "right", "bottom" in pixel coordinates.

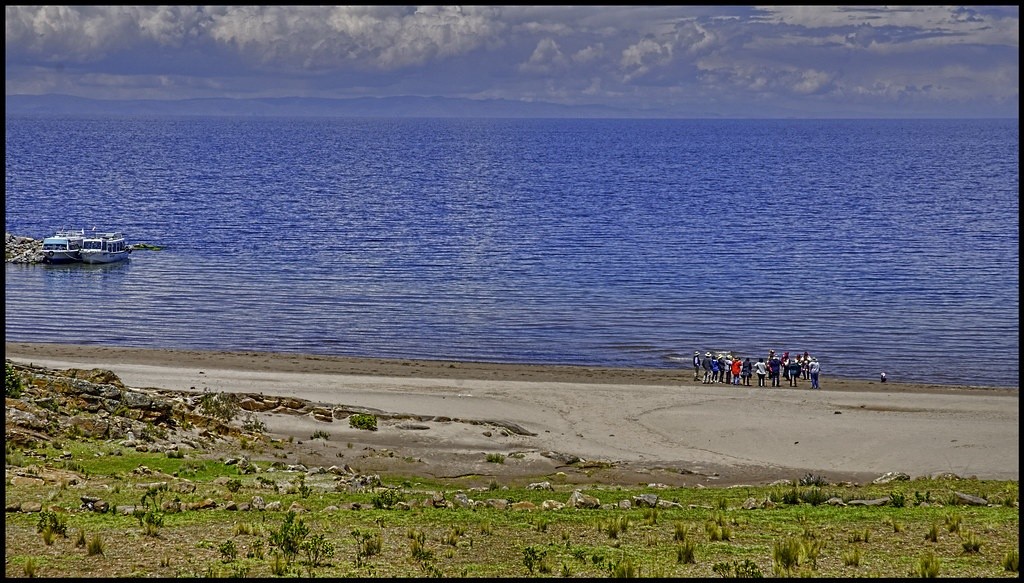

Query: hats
[
  {"left": 717, "top": 354, "right": 723, "bottom": 360},
  {"left": 694, "top": 351, "right": 700, "bottom": 356},
  {"left": 704, "top": 352, "right": 712, "bottom": 357}
]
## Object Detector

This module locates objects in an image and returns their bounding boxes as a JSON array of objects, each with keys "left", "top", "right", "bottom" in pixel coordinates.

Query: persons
[
  {"left": 693, "top": 350, "right": 820, "bottom": 389},
  {"left": 881, "top": 371, "right": 886, "bottom": 383}
]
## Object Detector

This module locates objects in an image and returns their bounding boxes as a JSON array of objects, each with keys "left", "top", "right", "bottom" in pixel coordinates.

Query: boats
[
  {"left": 77, "top": 231, "right": 133, "bottom": 265},
  {"left": 41, "top": 228, "right": 87, "bottom": 263}
]
